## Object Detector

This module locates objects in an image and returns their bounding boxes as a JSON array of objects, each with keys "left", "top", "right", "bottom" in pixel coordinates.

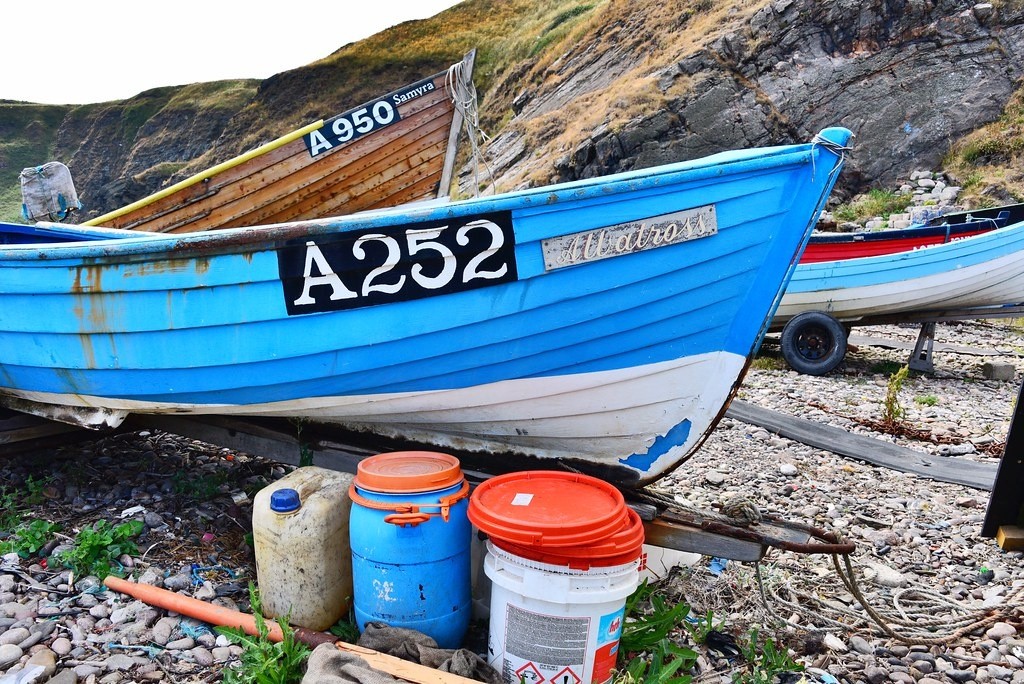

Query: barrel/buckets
[
  {"left": 484, "top": 539, "right": 641, "bottom": 684},
  {"left": 253, "top": 466, "right": 354, "bottom": 630},
  {"left": 346, "top": 452, "right": 471, "bottom": 649}
]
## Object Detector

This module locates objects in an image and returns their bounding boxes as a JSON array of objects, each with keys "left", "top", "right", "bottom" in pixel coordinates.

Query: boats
[
  {"left": 1, "top": 127, "right": 858, "bottom": 557},
  {"left": 78, "top": 44, "right": 477, "bottom": 234},
  {"left": 766, "top": 203, "right": 1024, "bottom": 337}
]
